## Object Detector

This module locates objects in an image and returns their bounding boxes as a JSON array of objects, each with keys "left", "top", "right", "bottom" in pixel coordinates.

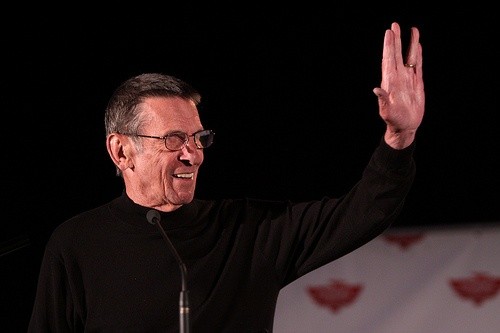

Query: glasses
[{"left": 120, "top": 130, "right": 217, "bottom": 152}]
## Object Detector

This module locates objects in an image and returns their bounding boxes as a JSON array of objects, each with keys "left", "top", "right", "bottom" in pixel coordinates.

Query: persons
[{"left": 28, "top": 24, "right": 425, "bottom": 333}]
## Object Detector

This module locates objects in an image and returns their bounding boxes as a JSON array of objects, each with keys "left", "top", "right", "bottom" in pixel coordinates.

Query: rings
[{"left": 404, "top": 64, "right": 415, "bottom": 68}]
